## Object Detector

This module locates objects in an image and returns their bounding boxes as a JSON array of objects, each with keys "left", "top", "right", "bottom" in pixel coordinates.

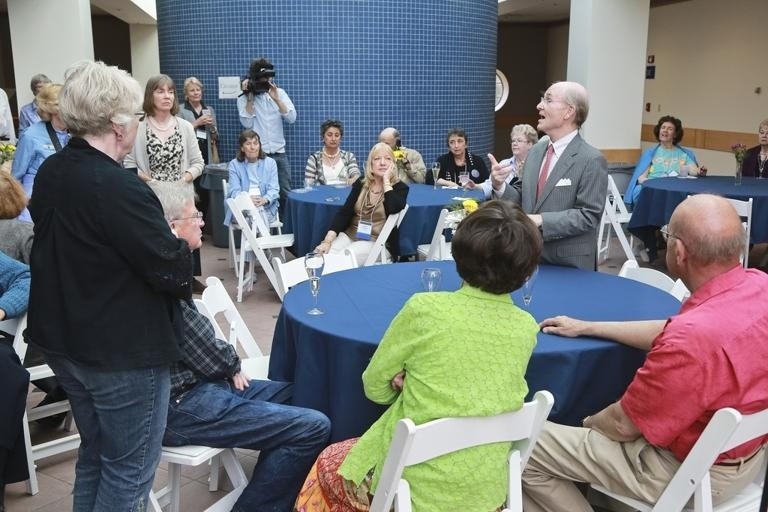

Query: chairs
[
  {"left": 268, "top": 244, "right": 361, "bottom": 293},
  {"left": 222, "top": 176, "right": 285, "bottom": 276},
  {"left": 191, "top": 273, "right": 278, "bottom": 383},
  {"left": 416, "top": 209, "right": 469, "bottom": 260},
  {"left": 1, "top": 304, "right": 94, "bottom": 499},
  {"left": 596, "top": 171, "right": 638, "bottom": 269},
  {"left": 346, "top": 196, "right": 410, "bottom": 270},
  {"left": 146, "top": 438, "right": 251, "bottom": 512},
  {"left": 225, "top": 191, "right": 295, "bottom": 303},
  {"left": 366, "top": 385, "right": 557, "bottom": 512},
  {"left": 686, "top": 192, "right": 755, "bottom": 271},
  {"left": 647, "top": 403, "right": 768, "bottom": 512},
  {"left": 617, "top": 254, "right": 691, "bottom": 303}
]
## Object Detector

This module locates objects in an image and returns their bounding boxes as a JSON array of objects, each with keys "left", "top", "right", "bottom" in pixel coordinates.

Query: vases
[{"left": 734, "top": 160, "right": 745, "bottom": 187}]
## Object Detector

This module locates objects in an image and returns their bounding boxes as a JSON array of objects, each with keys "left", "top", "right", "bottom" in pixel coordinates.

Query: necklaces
[{"left": 513, "top": 156, "right": 527, "bottom": 173}]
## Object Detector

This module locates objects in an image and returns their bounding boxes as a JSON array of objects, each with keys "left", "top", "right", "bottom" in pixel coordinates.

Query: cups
[
  {"left": 312, "top": 181, "right": 321, "bottom": 191},
  {"left": 680, "top": 165, "right": 688, "bottom": 178},
  {"left": 362, "top": 161, "right": 367, "bottom": 177},
  {"left": 422, "top": 268, "right": 441, "bottom": 292}
]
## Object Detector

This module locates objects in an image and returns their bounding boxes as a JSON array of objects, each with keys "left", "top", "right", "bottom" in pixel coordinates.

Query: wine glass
[
  {"left": 304, "top": 252, "right": 325, "bottom": 315},
  {"left": 431, "top": 162, "right": 440, "bottom": 190},
  {"left": 522, "top": 264, "right": 539, "bottom": 317},
  {"left": 459, "top": 172, "right": 469, "bottom": 199},
  {"left": 757, "top": 154, "right": 767, "bottom": 180}
]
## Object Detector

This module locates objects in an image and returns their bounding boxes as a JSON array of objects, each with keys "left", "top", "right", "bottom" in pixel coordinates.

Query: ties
[{"left": 537, "top": 145, "right": 554, "bottom": 199}]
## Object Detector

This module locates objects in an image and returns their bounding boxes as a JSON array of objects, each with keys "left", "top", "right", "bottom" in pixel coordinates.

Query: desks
[
  {"left": 629, "top": 173, "right": 763, "bottom": 253},
  {"left": 288, "top": 178, "right": 490, "bottom": 257},
  {"left": 263, "top": 255, "right": 688, "bottom": 441}
]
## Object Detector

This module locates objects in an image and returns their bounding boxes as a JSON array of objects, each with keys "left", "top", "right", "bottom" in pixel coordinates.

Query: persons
[
  {"left": 749, "top": 244, "right": 768, "bottom": 271},
  {"left": 236, "top": 58, "right": 299, "bottom": 233},
  {"left": 484, "top": 81, "right": 606, "bottom": 273},
  {"left": 623, "top": 116, "right": 708, "bottom": 265},
  {"left": 741, "top": 119, "right": 768, "bottom": 177},
  {"left": 1, "top": 74, "right": 73, "bottom": 485},
  {"left": 21, "top": 59, "right": 194, "bottom": 510},
  {"left": 223, "top": 132, "right": 281, "bottom": 283},
  {"left": 519, "top": 193, "right": 768, "bottom": 511},
  {"left": 460, "top": 122, "right": 537, "bottom": 195},
  {"left": 294, "top": 198, "right": 541, "bottom": 511},
  {"left": 282, "top": 120, "right": 488, "bottom": 286},
  {"left": 147, "top": 181, "right": 331, "bottom": 509},
  {"left": 123, "top": 72, "right": 219, "bottom": 293}
]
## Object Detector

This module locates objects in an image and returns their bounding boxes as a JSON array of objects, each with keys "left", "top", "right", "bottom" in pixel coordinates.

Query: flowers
[
  {"left": 462, "top": 198, "right": 479, "bottom": 214},
  {"left": 730, "top": 143, "right": 750, "bottom": 169}
]
